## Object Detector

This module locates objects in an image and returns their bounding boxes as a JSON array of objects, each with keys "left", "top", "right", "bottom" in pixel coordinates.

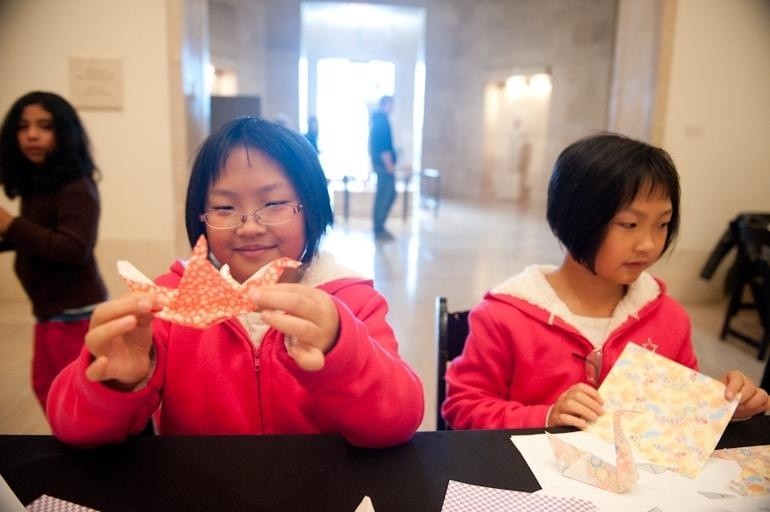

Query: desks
[{"left": 0, "top": 418, "right": 770, "bottom": 512}]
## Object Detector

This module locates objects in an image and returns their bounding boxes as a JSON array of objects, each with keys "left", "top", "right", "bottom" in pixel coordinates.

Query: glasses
[{"left": 197, "top": 202, "right": 304, "bottom": 230}]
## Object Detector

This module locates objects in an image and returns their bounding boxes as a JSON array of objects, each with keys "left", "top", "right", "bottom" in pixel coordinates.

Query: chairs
[{"left": 436, "top": 297, "right": 468, "bottom": 429}]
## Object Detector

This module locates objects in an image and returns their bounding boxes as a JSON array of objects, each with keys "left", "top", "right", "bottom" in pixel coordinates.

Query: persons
[
  {"left": 1, "top": 90, "right": 109, "bottom": 421},
  {"left": 368, "top": 95, "right": 401, "bottom": 242},
  {"left": 45, "top": 116, "right": 426, "bottom": 452},
  {"left": 438, "top": 127, "right": 769, "bottom": 433}
]
